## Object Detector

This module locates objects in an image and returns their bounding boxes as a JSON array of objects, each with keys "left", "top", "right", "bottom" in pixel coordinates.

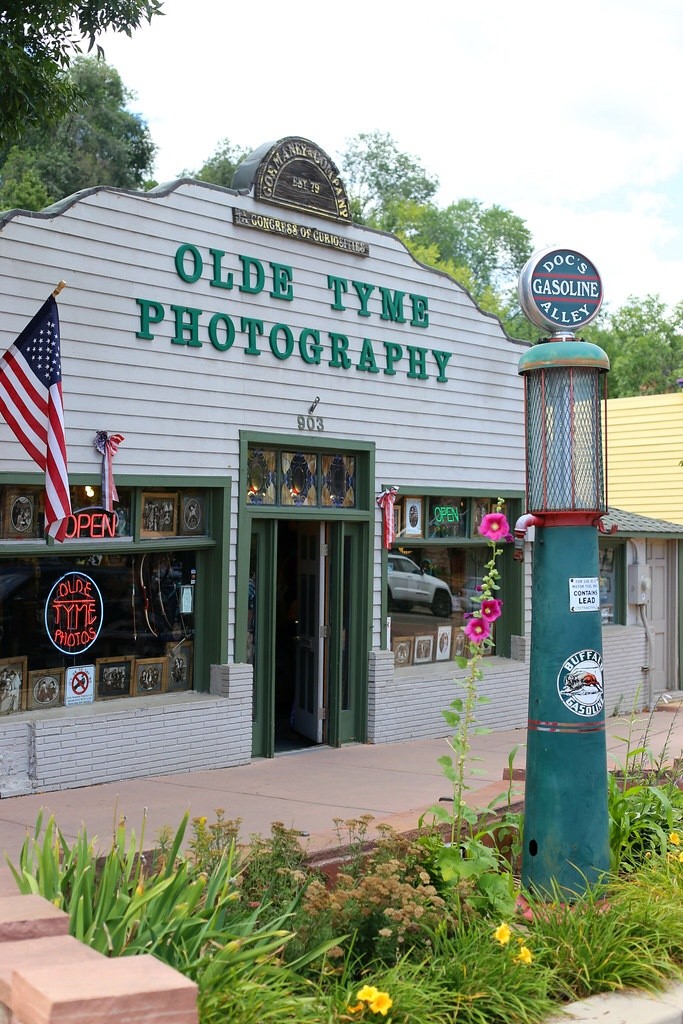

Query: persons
[
  {"left": 410, "top": 507, "right": 415, "bottom": 522},
  {"left": 0, "top": 668, "right": 21, "bottom": 711},
  {"left": 417, "top": 640, "right": 430, "bottom": 658},
  {"left": 171, "top": 657, "right": 183, "bottom": 682},
  {"left": 440, "top": 634, "right": 447, "bottom": 653},
  {"left": 13, "top": 500, "right": 30, "bottom": 530},
  {"left": 143, "top": 501, "right": 173, "bottom": 532},
  {"left": 103, "top": 669, "right": 125, "bottom": 689},
  {"left": 38, "top": 681, "right": 56, "bottom": 702},
  {"left": 474, "top": 505, "right": 487, "bottom": 535},
  {"left": 187, "top": 504, "right": 198, "bottom": 523},
  {"left": 398, "top": 646, "right": 407, "bottom": 661},
  {"left": 457, "top": 636, "right": 463, "bottom": 651},
  {"left": 141, "top": 668, "right": 158, "bottom": 690}
]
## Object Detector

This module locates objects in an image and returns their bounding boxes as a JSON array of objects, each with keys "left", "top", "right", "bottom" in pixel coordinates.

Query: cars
[
  {"left": 459, "top": 576, "right": 496, "bottom": 616},
  {"left": 388, "top": 553, "right": 453, "bottom": 618},
  {"left": 0, "top": 560, "right": 195, "bottom": 672}
]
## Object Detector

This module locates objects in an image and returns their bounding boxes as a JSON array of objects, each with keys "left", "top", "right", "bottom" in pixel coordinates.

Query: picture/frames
[
  {"left": 28, "top": 666, "right": 64, "bottom": 710},
  {"left": 491, "top": 504, "right": 507, "bottom": 517},
  {"left": 391, "top": 623, "right": 497, "bottom": 668},
  {"left": 139, "top": 491, "right": 178, "bottom": 538},
  {"left": 163, "top": 641, "right": 194, "bottom": 693},
  {"left": 403, "top": 495, "right": 425, "bottom": 538},
  {"left": 132, "top": 657, "right": 169, "bottom": 698},
  {"left": 96, "top": 655, "right": 135, "bottom": 703},
  {"left": 0, "top": 655, "right": 27, "bottom": 715},
  {"left": 393, "top": 504, "right": 401, "bottom": 538}
]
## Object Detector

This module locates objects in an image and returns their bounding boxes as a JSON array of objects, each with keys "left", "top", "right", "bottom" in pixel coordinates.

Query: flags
[{"left": 0, "top": 294, "right": 71, "bottom": 544}]
